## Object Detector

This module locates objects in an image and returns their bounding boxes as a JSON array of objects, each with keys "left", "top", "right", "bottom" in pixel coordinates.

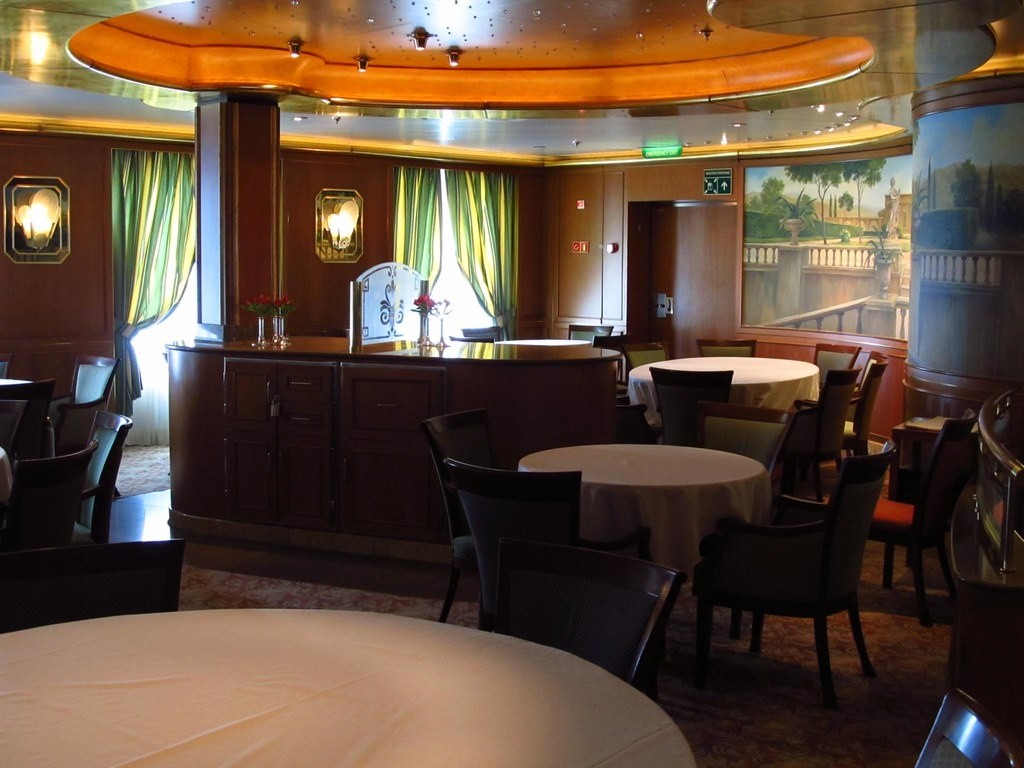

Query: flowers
[
  {"left": 247, "top": 294, "right": 298, "bottom": 317},
  {"left": 415, "top": 296, "right": 449, "bottom": 316}
]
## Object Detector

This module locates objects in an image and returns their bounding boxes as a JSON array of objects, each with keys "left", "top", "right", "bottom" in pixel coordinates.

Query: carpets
[
  {"left": 178, "top": 448, "right": 1024, "bottom": 768},
  {"left": 112, "top": 444, "right": 171, "bottom": 499}
]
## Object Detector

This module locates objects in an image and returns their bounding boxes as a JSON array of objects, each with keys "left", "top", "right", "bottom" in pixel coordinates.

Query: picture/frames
[{"left": 736, "top": 144, "right": 913, "bottom": 351}]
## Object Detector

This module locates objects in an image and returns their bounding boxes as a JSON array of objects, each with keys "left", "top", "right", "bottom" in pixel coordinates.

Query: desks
[
  {"left": 0, "top": 378, "right": 33, "bottom": 385},
  {"left": 518, "top": 444, "right": 771, "bottom": 570},
  {"left": 0, "top": 609, "right": 697, "bottom": 767},
  {"left": 496, "top": 340, "right": 591, "bottom": 346},
  {"left": 0, "top": 446, "right": 13, "bottom": 507},
  {"left": 628, "top": 356, "right": 820, "bottom": 432},
  {"left": 888, "top": 417, "right": 978, "bottom": 530}
]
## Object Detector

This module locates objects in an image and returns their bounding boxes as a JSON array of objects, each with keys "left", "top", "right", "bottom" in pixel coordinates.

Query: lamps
[
  {"left": 328, "top": 214, "right": 339, "bottom": 248},
  {"left": 356, "top": 54, "right": 370, "bottom": 72},
  {"left": 286, "top": 39, "right": 304, "bottom": 58},
  {"left": 337, "top": 201, "right": 359, "bottom": 248},
  {"left": 16, "top": 205, "right": 32, "bottom": 245},
  {"left": 444, "top": 49, "right": 463, "bottom": 66},
  {"left": 30, "top": 189, "right": 60, "bottom": 250},
  {"left": 408, "top": 33, "right": 432, "bottom": 51}
]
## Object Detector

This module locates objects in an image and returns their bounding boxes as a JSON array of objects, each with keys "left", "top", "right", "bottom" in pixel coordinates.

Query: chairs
[
  {"left": 0, "top": 354, "right": 186, "bottom": 636},
  {"left": 420, "top": 325, "right": 1024, "bottom": 768}
]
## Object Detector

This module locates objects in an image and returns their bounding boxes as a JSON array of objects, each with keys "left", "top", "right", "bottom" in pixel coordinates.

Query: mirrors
[
  {"left": 2, "top": 176, "right": 71, "bottom": 263},
  {"left": 314, "top": 188, "right": 364, "bottom": 263}
]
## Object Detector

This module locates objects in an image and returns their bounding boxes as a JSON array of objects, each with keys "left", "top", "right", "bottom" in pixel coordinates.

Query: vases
[
  {"left": 251, "top": 317, "right": 289, "bottom": 348},
  {"left": 418, "top": 313, "right": 450, "bottom": 348}
]
